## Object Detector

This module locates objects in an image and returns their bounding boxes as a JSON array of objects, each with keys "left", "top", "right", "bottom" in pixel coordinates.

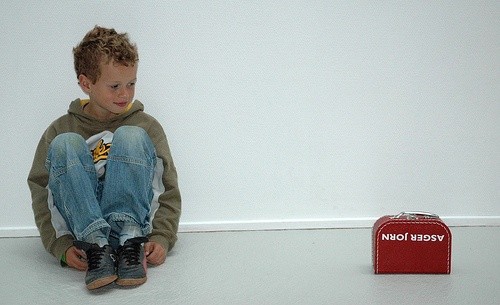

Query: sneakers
[
  {"left": 73, "top": 240, "right": 119, "bottom": 290},
  {"left": 116, "top": 237, "right": 148, "bottom": 285}
]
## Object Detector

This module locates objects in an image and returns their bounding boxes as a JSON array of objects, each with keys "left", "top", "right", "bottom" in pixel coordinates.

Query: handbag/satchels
[{"left": 372, "top": 211, "right": 451, "bottom": 273}]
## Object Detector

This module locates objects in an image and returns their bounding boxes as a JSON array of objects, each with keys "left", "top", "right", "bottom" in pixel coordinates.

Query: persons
[{"left": 27, "top": 25, "right": 181, "bottom": 290}]
